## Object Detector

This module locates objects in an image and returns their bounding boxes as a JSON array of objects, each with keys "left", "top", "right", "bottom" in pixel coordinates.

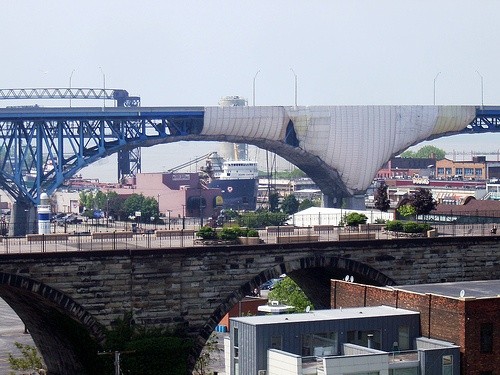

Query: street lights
[
  {"left": 69, "top": 68, "right": 74, "bottom": 107},
  {"left": 475, "top": 70, "right": 483, "bottom": 109},
  {"left": 251, "top": 69, "right": 260, "bottom": 107},
  {"left": 433, "top": 71, "right": 439, "bottom": 106},
  {"left": 290, "top": 67, "right": 298, "bottom": 110},
  {"left": 99, "top": 67, "right": 106, "bottom": 108}
]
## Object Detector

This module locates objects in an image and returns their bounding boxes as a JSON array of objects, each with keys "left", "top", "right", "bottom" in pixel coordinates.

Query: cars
[{"left": 51, "top": 213, "right": 88, "bottom": 226}]
